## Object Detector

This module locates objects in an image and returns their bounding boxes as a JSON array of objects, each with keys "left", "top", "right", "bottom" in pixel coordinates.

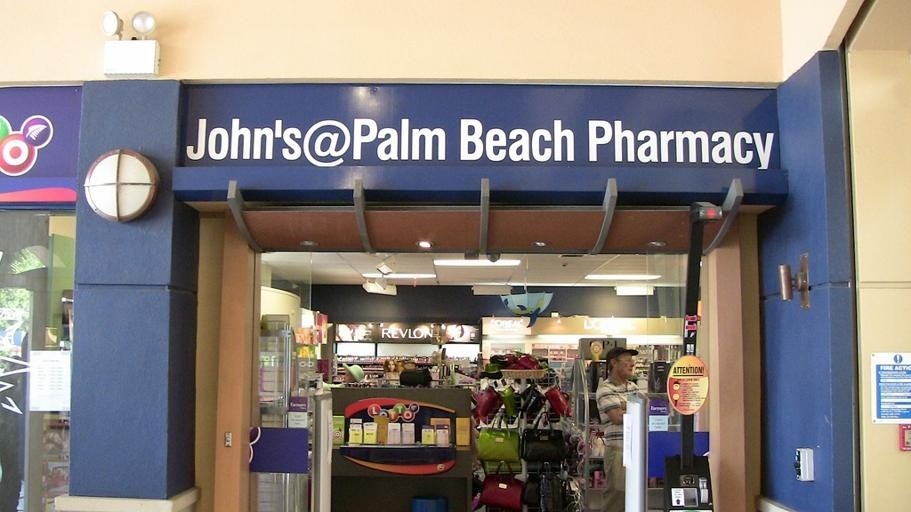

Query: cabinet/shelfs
[{"left": 534, "top": 343, "right": 682, "bottom": 509}]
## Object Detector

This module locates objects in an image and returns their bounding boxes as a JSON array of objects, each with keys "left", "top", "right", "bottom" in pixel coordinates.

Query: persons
[
  {"left": 597, "top": 346, "right": 640, "bottom": 512},
  {"left": 383, "top": 358, "right": 398, "bottom": 373}
]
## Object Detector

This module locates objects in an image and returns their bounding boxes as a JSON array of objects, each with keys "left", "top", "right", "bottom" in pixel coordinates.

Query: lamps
[
  {"left": 374, "top": 259, "right": 394, "bottom": 291},
  {"left": 95, "top": 7, "right": 162, "bottom": 80},
  {"left": 775, "top": 252, "right": 810, "bottom": 308},
  {"left": 81, "top": 146, "right": 161, "bottom": 226}
]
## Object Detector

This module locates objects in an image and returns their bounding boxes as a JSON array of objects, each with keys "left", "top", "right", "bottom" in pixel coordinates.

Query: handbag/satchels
[
  {"left": 400, "top": 362, "right": 432, "bottom": 386},
  {"left": 485, "top": 352, "right": 547, "bottom": 372},
  {"left": 476, "top": 384, "right": 573, "bottom": 424},
  {"left": 476, "top": 426, "right": 572, "bottom": 512}
]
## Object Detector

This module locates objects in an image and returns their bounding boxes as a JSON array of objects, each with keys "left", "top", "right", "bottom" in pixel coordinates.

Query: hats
[{"left": 606, "top": 347, "right": 639, "bottom": 360}]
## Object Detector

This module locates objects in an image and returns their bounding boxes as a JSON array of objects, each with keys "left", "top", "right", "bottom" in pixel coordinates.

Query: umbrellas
[{"left": 500, "top": 260, "right": 555, "bottom": 328}]
